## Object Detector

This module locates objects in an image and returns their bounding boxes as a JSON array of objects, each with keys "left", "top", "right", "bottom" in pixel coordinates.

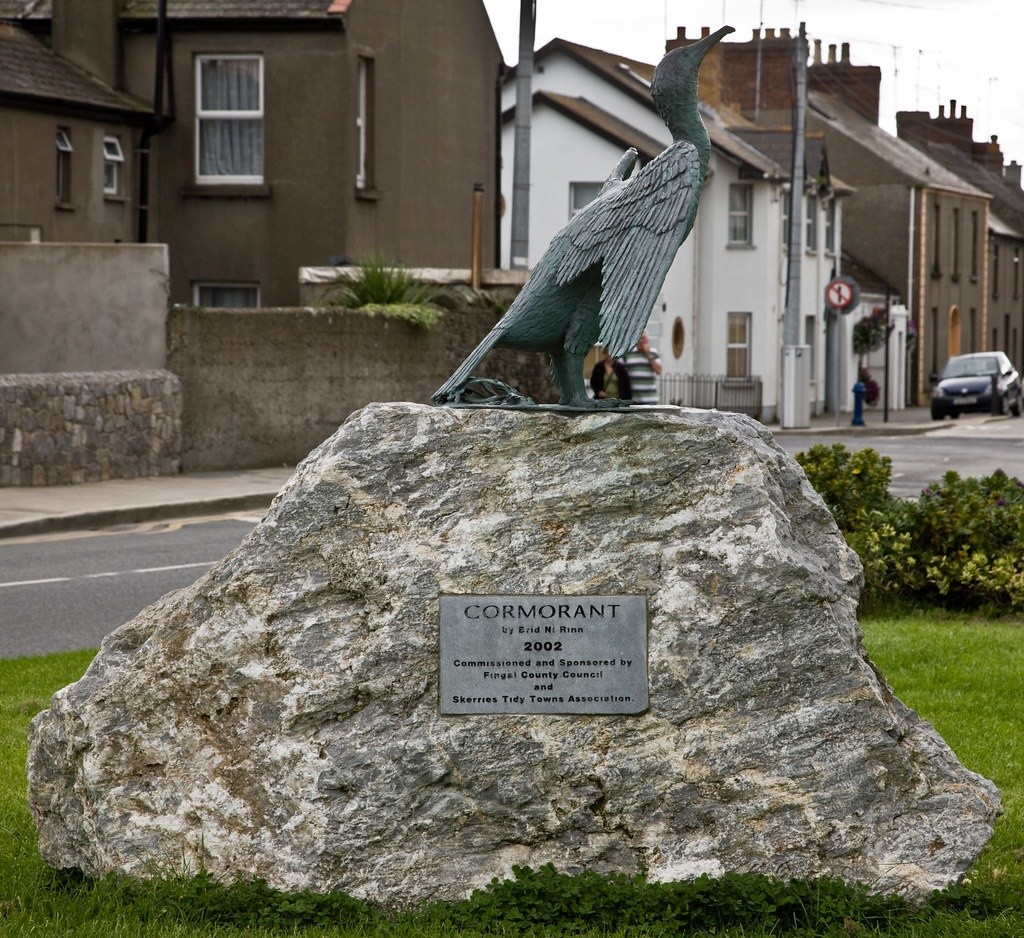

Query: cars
[{"left": 931, "top": 351, "right": 1024, "bottom": 421}]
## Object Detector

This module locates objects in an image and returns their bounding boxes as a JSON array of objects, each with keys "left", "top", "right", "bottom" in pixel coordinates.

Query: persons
[{"left": 590, "top": 329, "right": 664, "bottom": 405}]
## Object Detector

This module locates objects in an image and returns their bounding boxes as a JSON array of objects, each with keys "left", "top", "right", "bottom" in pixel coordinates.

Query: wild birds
[{"left": 428, "top": 24, "right": 734, "bottom": 400}]
